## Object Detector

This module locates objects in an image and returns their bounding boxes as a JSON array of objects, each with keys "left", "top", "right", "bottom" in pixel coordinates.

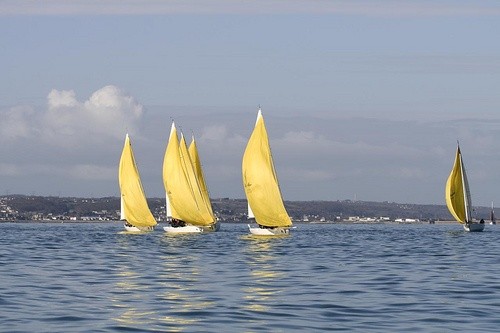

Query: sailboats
[
  {"left": 162, "top": 119, "right": 221, "bottom": 233},
  {"left": 241, "top": 105, "right": 297, "bottom": 236},
  {"left": 118, "top": 133, "right": 158, "bottom": 232},
  {"left": 445, "top": 138, "right": 496, "bottom": 232}
]
